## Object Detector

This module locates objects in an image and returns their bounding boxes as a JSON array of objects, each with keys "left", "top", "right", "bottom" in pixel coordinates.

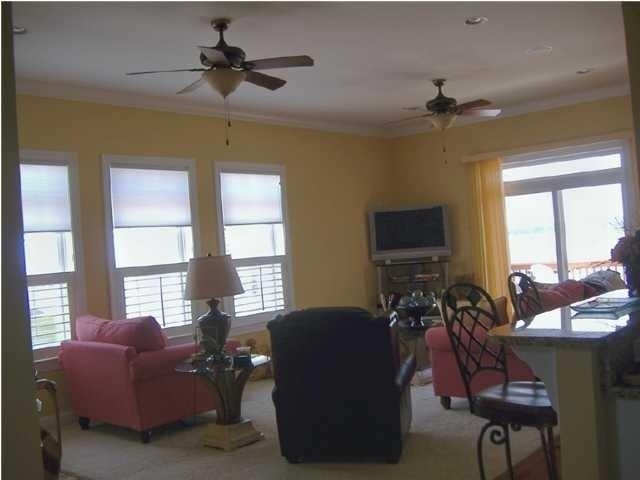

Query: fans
[
  {"left": 387, "top": 78, "right": 502, "bottom": 124},
  {"left": 126, "top": 19, "right": 314, "bottom": 96}
]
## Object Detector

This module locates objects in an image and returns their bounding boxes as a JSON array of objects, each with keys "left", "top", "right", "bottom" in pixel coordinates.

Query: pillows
[
  {"left": 433, "top": 295, "right": 509, "bottom": 328},
  {"left": 75, "top": 314, "right": 168, "bottom": 352}
]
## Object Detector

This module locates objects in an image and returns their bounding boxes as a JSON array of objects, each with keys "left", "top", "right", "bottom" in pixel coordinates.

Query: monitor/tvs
[{"left": 369, "top": 200, "right": 451, "bottom": 264}]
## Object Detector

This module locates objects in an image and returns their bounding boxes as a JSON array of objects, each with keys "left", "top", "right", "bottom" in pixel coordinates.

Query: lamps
[
  {"left": 430, "top": 112, "right": 455, "bottom": 165},
  {"left": 184, "top": 252, "right": 246, "bottom": 362},
  {"left": 202, "top": 66, "right": 244, "bottom": 148}
]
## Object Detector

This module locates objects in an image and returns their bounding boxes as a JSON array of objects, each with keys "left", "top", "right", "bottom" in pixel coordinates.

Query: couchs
[{"left": 424, "top": 268, "right": 625, "bottom": 409}]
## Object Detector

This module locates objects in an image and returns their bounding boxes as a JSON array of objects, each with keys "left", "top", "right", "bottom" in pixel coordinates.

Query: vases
[{"left": 622, "top": 259, "right": 640, "bottom": 298}]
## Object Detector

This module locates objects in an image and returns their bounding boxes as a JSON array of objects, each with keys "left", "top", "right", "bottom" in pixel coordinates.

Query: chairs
[
  {"left": 440, "top": 282, "right": 558, "bottom": 479},
  {"left": 266, "top": 304, "right": 417, "bottom": 465},
  {"left": 59, "top": 338, "right": 244, "bottom": 444},
  {"left": 507, "top": 271, "right": 545, "bottom": 320}
]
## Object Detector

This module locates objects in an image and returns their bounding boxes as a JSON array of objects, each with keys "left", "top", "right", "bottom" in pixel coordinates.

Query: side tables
[
  {"left": 396, "top": 315, "right": 443, "bottom": 387},
  {"left": 174, "top": 354, "right": 272, "bottom": 452}
]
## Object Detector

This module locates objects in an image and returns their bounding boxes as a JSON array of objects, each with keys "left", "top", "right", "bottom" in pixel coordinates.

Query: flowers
[{"left": 608, "top": 215, "right": 640, "bottom": 262}]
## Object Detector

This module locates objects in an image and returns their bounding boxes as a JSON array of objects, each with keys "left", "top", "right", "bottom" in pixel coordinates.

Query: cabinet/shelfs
[{"left": 375, "top": 259, "right": 451, "bottom": 309}]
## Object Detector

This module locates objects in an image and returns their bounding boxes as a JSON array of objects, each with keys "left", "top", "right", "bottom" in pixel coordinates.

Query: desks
[{"left": 487, "top": 288, "right": 640, "bottom": 480}]
